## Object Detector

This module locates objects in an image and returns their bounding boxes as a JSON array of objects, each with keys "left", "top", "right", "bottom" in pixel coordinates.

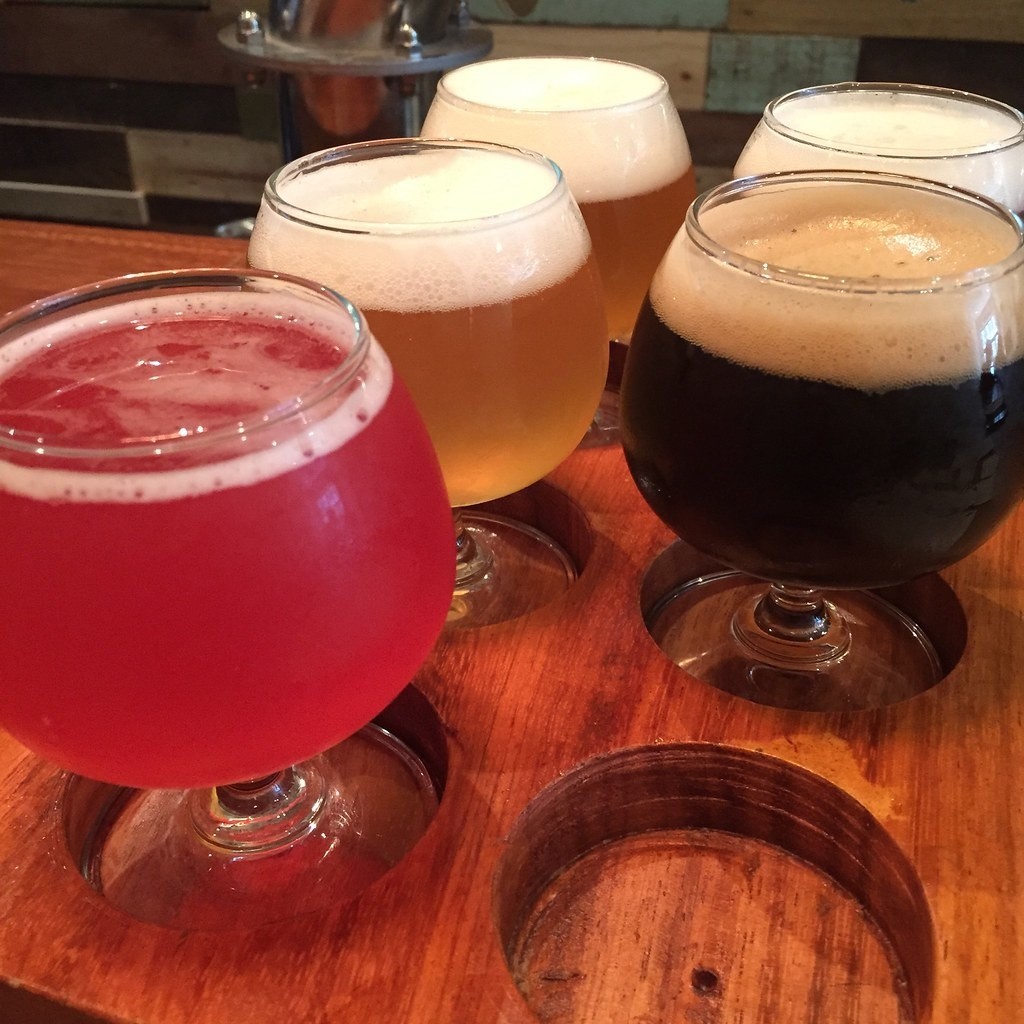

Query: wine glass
[
  {"left": 0, "top": 269, "right": 459, "bottom": 935},
  {"left": 626, "top": 174, "right": 1024, "bottom": 713},
  {"left": 419, "top": 55, "right": 695, "bottom": 447},
  {"left": 735, "top": 82, "right": 1024, "bottom": 223},
  {"left": 247, "top": 138, "right": 610, "bottom": 629}
]
